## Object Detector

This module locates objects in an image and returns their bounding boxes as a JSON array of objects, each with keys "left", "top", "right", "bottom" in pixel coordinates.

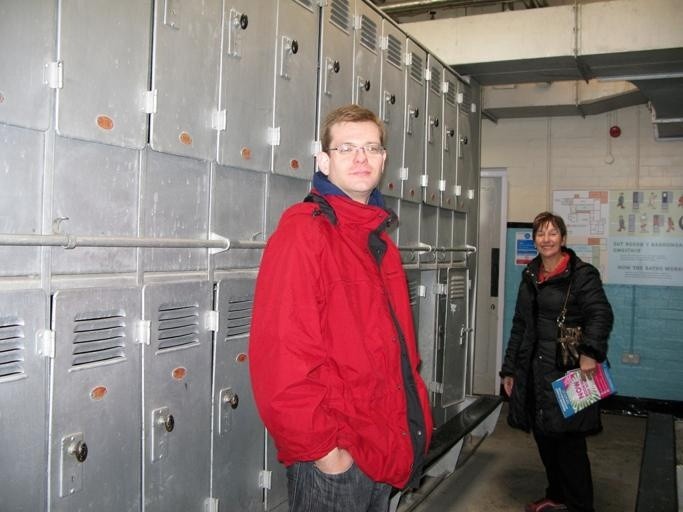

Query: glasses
[{"left": 326, "top": 144, "right": 387, "bottom": 155}]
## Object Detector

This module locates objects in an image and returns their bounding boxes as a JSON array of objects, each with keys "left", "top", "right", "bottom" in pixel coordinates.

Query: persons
[
  {"left": 246, "top": 104, "right": 435, "bottom": 511},
  {"left": 497, "top": 211, "right": 613, "bottom": 511}
]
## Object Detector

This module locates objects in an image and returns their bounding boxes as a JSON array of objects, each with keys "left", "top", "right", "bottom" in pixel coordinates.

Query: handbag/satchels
[{"left": 556, "top": 325, "right": 611, "bottom": 371}]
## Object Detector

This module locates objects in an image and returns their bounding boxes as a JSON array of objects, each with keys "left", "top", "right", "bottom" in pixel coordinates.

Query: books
[{"left": 549, "top": 359, "right": 618, "bottom": 420}]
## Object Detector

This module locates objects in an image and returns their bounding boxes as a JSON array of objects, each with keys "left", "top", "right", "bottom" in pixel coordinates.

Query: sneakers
[{"left": 525, "top": 496, "right": 567, "bottom": 511}]
